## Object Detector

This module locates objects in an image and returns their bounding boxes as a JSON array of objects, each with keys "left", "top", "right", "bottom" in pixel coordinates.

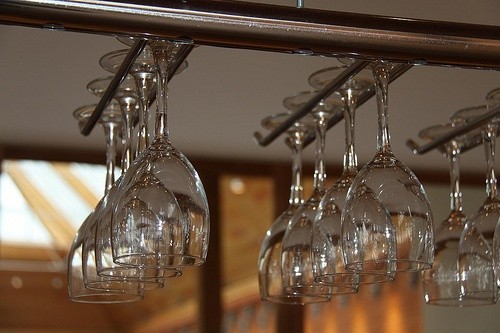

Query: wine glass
[
  {"left": 66, "top": 32, "right": 210, "bottom": 305},
  {"left": 257, "top": 53, "right": 500, "bottom": 308}
]
